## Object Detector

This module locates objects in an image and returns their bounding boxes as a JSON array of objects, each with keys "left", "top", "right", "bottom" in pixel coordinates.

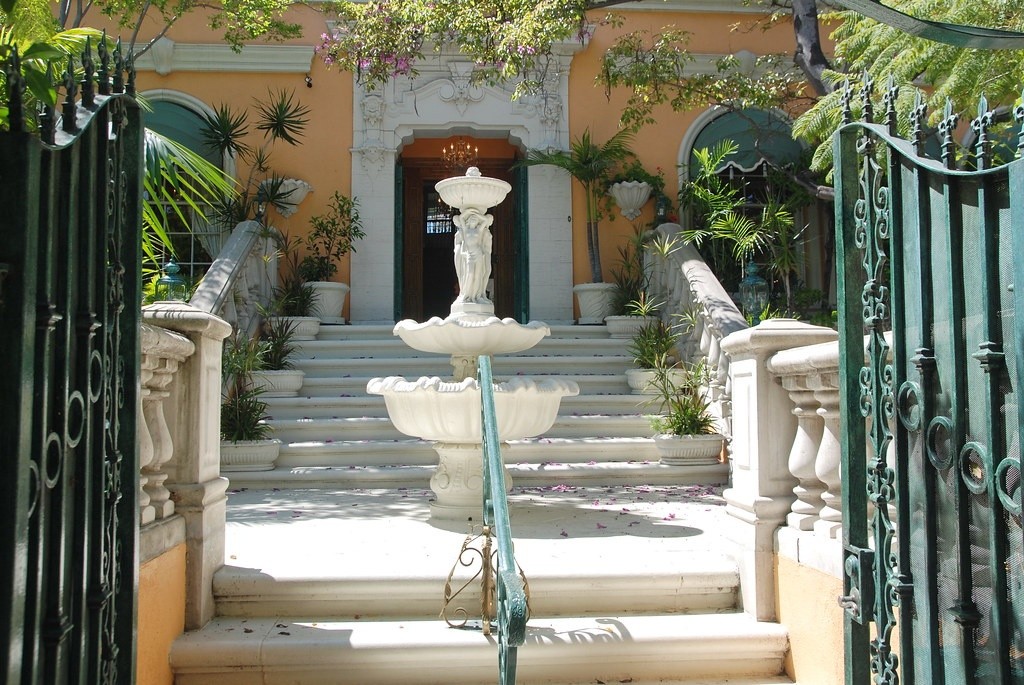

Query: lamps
[{"left": 442, "top": 135, "right": 480, "bottom": 174}]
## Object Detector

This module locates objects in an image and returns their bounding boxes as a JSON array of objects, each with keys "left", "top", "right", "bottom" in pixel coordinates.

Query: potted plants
[{"left": 219, "top": 125, "right": 726, "bottom": 471}]
